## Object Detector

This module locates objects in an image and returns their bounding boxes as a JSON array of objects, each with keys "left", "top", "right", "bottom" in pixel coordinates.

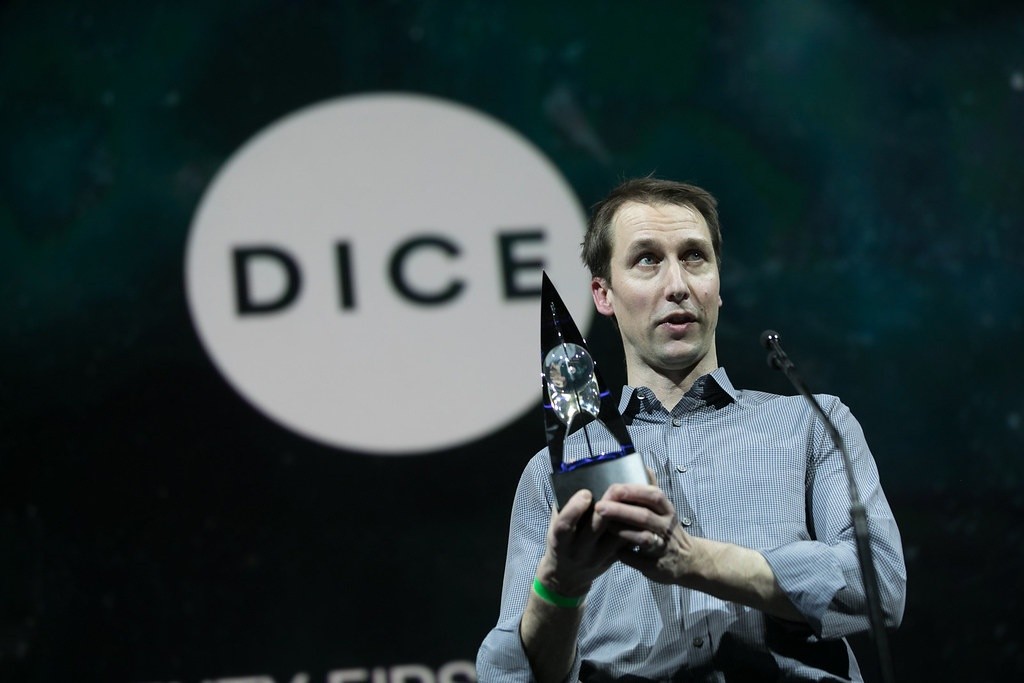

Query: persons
[{"left": 476, "top": 178, "right": 905, "bottom": 683}]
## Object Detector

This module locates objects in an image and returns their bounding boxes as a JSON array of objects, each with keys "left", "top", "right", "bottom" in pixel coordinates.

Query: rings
[{"left": 632, "top": 545, "right": 641, "bottom": 555}]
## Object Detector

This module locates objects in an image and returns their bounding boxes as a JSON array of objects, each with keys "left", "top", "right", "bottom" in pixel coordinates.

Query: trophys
[{"left": 540, "top": 270, "right": 649, "bottom": 515}]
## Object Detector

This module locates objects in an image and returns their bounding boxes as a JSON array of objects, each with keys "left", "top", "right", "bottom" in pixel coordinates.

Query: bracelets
[{"left": 533, "top": 578, "right": 587, "bottom": 609}]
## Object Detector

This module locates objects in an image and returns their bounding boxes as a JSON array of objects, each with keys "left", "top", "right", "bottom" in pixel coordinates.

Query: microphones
[{"left": 761, "top": 328, "right": 859, "bottom": 506}]
